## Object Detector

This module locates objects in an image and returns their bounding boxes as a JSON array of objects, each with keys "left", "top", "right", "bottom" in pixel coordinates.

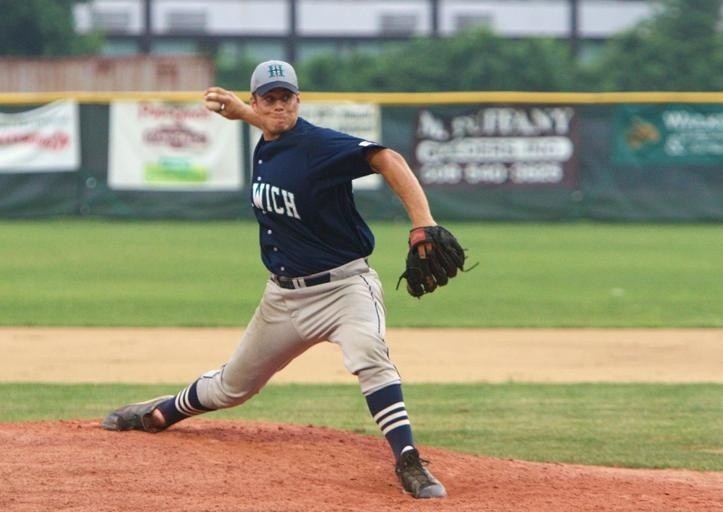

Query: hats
[{"left": 250, "top": 60, "right": 298, "bottom": 97}]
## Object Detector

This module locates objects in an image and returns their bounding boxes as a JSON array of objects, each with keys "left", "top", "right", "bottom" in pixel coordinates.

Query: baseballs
[{"left": 206, "top": 92, "right": 223, "bottom": 111}]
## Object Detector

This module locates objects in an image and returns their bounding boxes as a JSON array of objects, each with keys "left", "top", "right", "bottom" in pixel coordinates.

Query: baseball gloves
[{"left": 395, "top": 225, "right": 476, "bottom": 297}]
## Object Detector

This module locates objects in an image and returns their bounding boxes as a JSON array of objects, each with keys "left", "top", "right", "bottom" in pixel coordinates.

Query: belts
[{"left": 270, "top": 272, "right": 331, "bottom": 289}]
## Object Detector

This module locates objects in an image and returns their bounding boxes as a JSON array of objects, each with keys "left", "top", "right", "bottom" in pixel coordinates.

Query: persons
[{"left": 102, "top": 59, "right": 464, "bottom": 500}]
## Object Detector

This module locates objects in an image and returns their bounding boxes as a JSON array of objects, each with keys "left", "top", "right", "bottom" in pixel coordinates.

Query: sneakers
[
  {"left": 395, "top": 448, "right": 447, "bottom": 499},
  {"left": 102, "top": 394, "right": 175, "bottom": 433}
]
